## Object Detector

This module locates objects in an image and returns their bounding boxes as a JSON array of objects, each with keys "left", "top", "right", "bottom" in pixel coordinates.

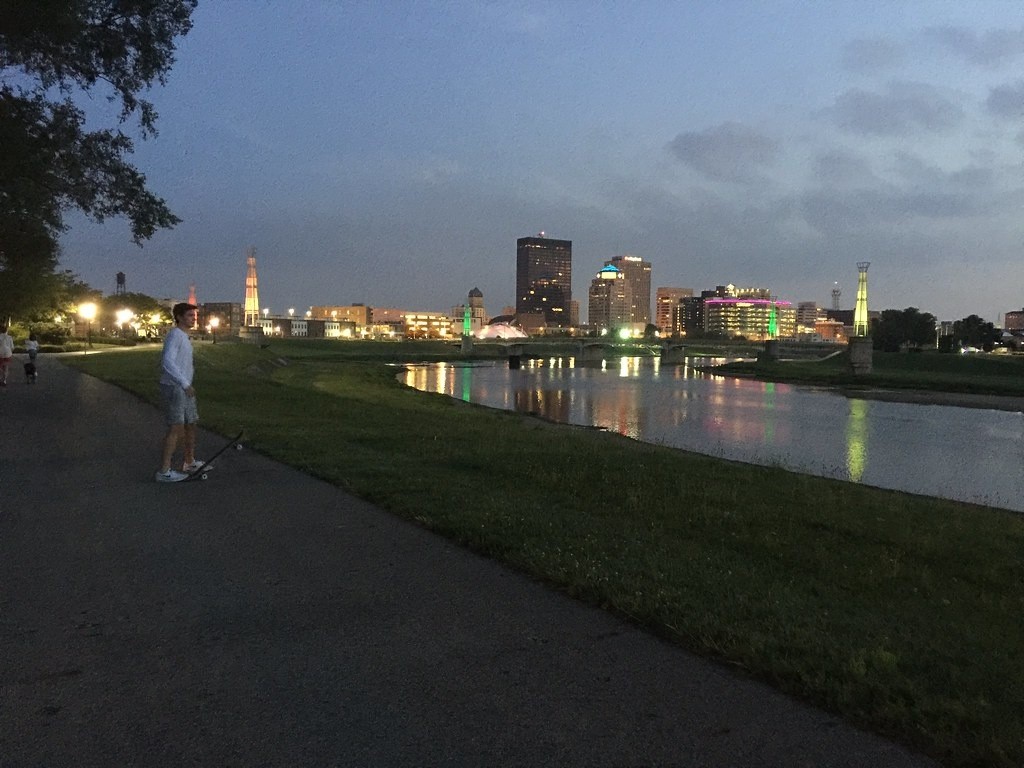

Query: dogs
[{"left": 23, "top": 361, "right": 37, "bottom": 385}]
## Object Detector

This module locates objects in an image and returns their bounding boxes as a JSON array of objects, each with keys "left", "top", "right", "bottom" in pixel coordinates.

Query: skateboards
[{"left": 184, "top": 429, "right": 247, "bottom": 481}]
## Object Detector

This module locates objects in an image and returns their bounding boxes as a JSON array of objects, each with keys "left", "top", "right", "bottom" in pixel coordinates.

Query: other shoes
[{"left": 1, "top": 379, "right": 6, "bottom": 385}]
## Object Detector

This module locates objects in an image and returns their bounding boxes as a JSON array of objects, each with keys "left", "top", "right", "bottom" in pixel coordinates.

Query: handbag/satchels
[{"left": 142, "top": 378, "right": 177, "bottom": 407}]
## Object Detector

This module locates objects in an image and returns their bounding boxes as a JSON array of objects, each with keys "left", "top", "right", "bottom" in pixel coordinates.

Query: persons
[
  {"left": 27, "top": 332, "right": 39, "bottom": 364},
  {"left": 153, "top": 302, "right": 213, "bottom": 483},
  {"left": 0, "top": 325, "right": 16, "bottom": 386}
]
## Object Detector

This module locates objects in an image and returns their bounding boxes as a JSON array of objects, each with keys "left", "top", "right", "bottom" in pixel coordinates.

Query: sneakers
[
  {"left": 182, "top": 462, "right": 214, "bottom": 474},
  {"left": 155, "top": 469, "right": 190, "bottom": 483}
]
